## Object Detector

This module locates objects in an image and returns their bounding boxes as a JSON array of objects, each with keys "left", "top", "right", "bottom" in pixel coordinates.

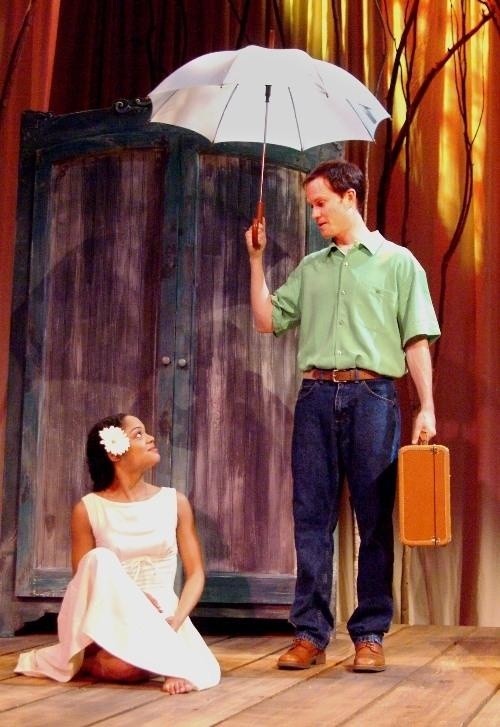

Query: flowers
[{"left": 98, "top": 424, "right": 131, "bottom": 455}]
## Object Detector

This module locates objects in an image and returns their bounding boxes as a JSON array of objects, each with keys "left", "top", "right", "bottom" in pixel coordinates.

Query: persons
[
  {"left": 11, "top": 411, "right": 224, "bottom": 697},
  {"left": 245, "top": 156, "right": 445, "bottom": 673}
]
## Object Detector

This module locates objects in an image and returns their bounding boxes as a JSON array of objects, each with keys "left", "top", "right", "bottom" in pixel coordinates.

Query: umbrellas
[{"left": 144, "top": 42, "right": 396, "bottom": 251}]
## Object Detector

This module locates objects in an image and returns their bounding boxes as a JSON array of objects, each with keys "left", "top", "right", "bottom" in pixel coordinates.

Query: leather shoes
[
  {"left": 351, "top": 636, "right": 387, "bottom": 672},
  {"left": 277, "top": 634, "right": 327, "bottom": 669}
]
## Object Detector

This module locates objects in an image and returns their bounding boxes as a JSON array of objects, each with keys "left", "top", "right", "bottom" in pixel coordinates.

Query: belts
[{"left": 301, "top": 365, "right": 386, "bottom": 382}]
